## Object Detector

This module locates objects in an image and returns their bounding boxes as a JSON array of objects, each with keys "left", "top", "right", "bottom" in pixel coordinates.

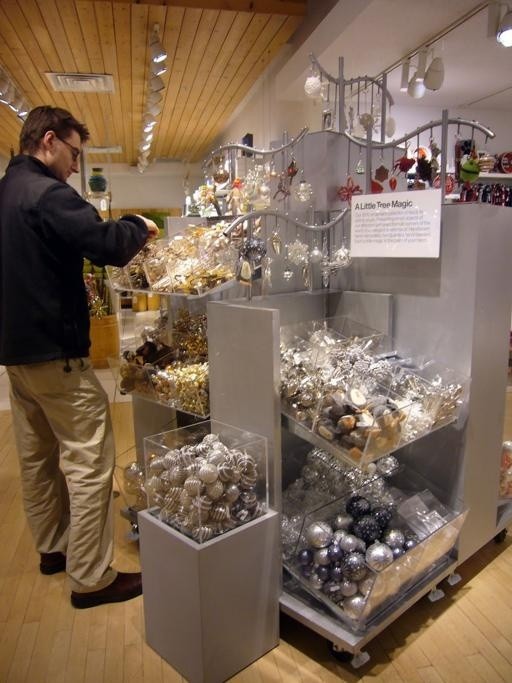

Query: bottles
[
  {"left": 88, "top": 166, "right": 107, "bottom": 191},
  {"left": 459, "top": 183, "right": 511, "bottom": 206}
]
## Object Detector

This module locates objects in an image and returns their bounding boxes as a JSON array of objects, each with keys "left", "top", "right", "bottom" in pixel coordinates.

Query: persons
[{"left": 0, "top": 105, "right": 160, "bottom": 609}]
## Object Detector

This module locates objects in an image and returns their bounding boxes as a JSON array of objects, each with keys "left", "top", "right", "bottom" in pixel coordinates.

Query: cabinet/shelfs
[{"left": 108, "top": 267, "right": 459, "bottom": 666}]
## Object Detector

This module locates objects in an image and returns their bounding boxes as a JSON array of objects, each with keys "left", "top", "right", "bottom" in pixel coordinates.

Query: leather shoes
[
  {"left": 39, "top": 552, "right": 67, "bottom": 574},
  {"left": 70, "top": 571, "right": 143, "bottom": 608}
]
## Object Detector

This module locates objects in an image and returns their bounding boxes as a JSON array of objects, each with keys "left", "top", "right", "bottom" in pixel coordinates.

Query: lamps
[
  {"left": 1, "top": 71, "right": 34, "bottom": 121},
  {"left": 134, "top": 22, "right": 170, "bottom": 174},
  {"left": 321, "top": 1, "right": 512, "bottom": 117}
]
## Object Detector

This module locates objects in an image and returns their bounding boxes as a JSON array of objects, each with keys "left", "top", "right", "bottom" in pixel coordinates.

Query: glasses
[{"left": 58, "top": 135, "right": 81, "bottom": 159}]
